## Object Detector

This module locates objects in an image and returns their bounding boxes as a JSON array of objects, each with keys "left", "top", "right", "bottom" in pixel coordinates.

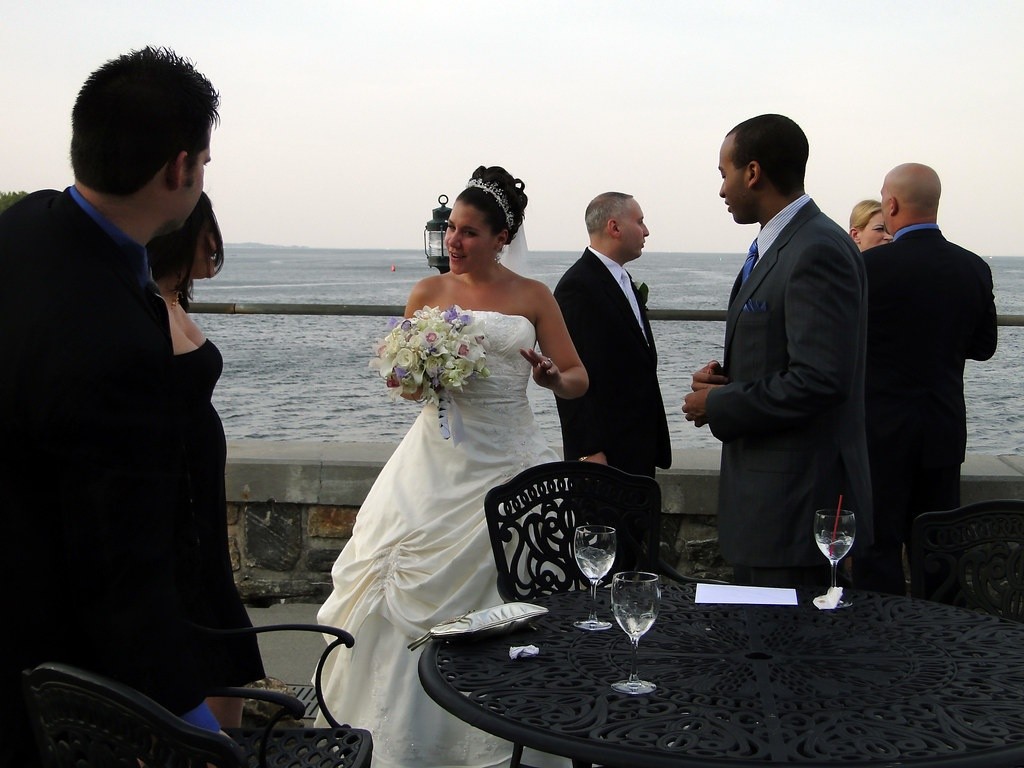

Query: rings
[{"left": 547, "top": 358, "right": 552, "bottom": 364}]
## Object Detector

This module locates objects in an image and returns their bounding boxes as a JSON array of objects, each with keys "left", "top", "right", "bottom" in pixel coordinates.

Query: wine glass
[
  {"left": 574, "top": 525, "right": 617, "bottom": 630},
  {"left": 812, "top": 509, "right": 857, "bottom": 608},
  {"left": 611, "top": 571, "right": 661, "bottom": 695}
]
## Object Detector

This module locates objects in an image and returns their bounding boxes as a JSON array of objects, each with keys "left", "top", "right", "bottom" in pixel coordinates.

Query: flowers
[{"left": 367, "top": 305, "right": 489, "bottom": 440}]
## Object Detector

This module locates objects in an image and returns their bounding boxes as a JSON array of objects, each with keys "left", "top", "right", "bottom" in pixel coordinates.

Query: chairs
[
  {"left": 21, "top": 613, "right": 373, "bottom": 768},
  {"left": 484, "top": 461, "right": 733, "bottom": 768},
  {"left": 907, "top": 500, "right": 1024, "bottom": 623}
]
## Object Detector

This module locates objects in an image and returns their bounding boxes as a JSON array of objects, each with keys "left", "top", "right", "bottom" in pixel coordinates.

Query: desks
[{"left": 418, "top": 579, "right": 1024, "bottom": 768}]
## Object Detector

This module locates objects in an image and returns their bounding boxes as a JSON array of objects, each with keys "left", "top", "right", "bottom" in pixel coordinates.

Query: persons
[
  {"left": 0, "top": 40, "right": 264, "bottom": 768},
  {"left": 545, "top": 192, "right": 674, "bottom": 580},
  {"left": 315, "top": 166, "right": 593, "bottom": 768},
  {"left": 849, "top": 162, "right": 997, "bottom": 599},
  {"left": 682, "top": 114, "right": 862, "bottom": 594}
]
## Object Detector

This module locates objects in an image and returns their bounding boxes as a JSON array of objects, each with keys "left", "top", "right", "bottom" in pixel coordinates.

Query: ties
[{"left": 741, "top": 238, "right": 758, "bottom": 290}]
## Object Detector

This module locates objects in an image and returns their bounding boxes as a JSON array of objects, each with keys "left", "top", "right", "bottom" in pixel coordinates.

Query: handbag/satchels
[{"left": 408, "top": 602, "right": 548, "bottom": 651}]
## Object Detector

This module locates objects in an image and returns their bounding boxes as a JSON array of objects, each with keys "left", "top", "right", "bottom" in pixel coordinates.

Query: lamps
[{"left": 424, "top": 194, "right": 453, "bottom": 274}]
